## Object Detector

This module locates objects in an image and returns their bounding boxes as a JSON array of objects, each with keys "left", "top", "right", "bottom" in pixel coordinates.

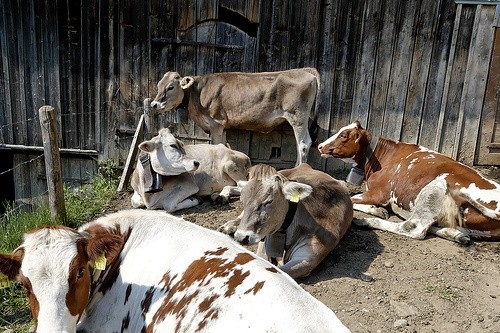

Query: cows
[
  {"left": 317, "top": 120, "right": 500, "bottom": 245},
  {"left": 149, "top": 70, "right": 322, "bottom": 167},
  {"left": 0, "top": 207, "right": 351, "bottom": 333},
  {"left": 130, "top": 128, "right": 354, "bottom": 280}
]
